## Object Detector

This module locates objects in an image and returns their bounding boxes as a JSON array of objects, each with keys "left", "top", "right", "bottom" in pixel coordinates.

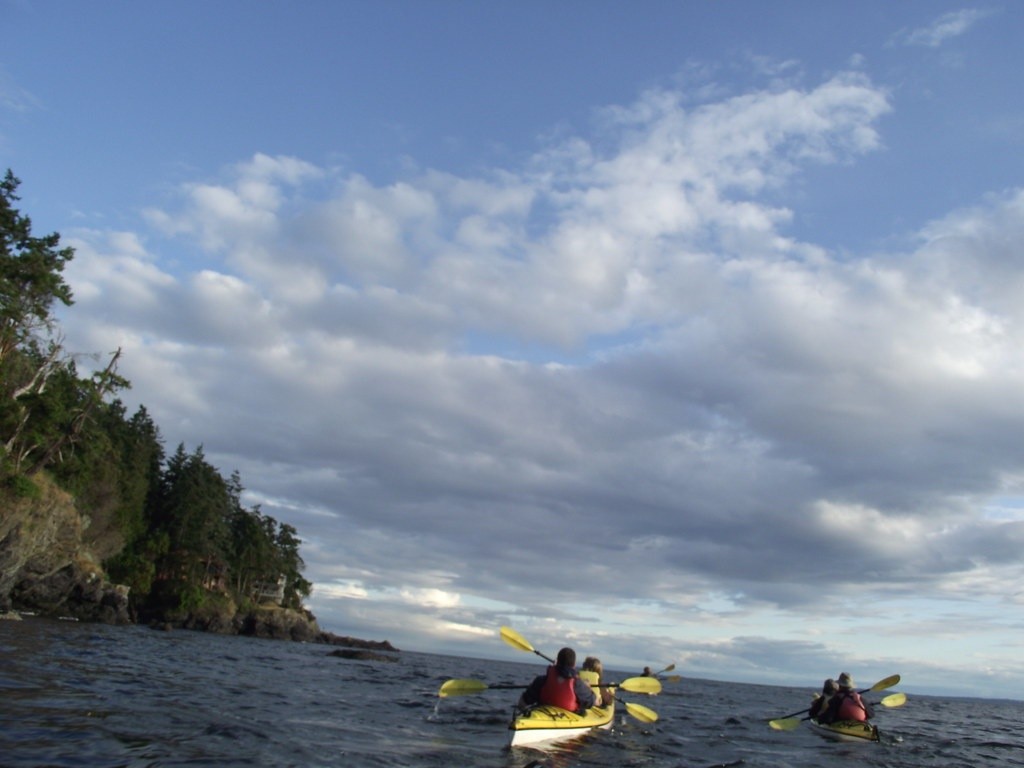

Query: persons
[
  {"left": 809, "top": 672, "right": 866, "bottom": 724},
  {"left": 523, "top": 647, "right": 595, "bottom": 715},
  {"left": 640, "top": 666, "right": 657, "bottom": 677},
  {"left": 577, "top": 656, "right": 611, "bottom": 709}
]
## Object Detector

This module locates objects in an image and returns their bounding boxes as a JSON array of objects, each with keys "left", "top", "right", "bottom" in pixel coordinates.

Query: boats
[
  {"left": 506, "top": 681, "right": 616, "bottom": 746},
  {"left": 809, "top": 692, "right": 879, "bottom": 743}
]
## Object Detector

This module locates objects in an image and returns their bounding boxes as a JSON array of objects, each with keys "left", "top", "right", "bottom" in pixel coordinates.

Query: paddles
[
  {"left": 761, "top": 674, "right": 901, "bottom": 721},
  {"left": 657, "top": 676, "right": 681, "bottom": 681},
  {"left": 437, "top": 677, "right": 662, "bottom": 698},
  {"left": 769, "top": 693, "right": 907, "bottom": 731},
  {"left": 651, "top": 664, "right": 675, "bottom": 676},
  {"left": 500, "top": 625, "right": 660, "bottom": 723}
]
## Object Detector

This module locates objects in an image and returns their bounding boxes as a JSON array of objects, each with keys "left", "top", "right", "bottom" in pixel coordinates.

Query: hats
[
  {"left": 824, "top": 678, "right": 838, "bottom": 690},
  {"left": 834, "top": 672, "right": 858, "bottom": 688}
]
